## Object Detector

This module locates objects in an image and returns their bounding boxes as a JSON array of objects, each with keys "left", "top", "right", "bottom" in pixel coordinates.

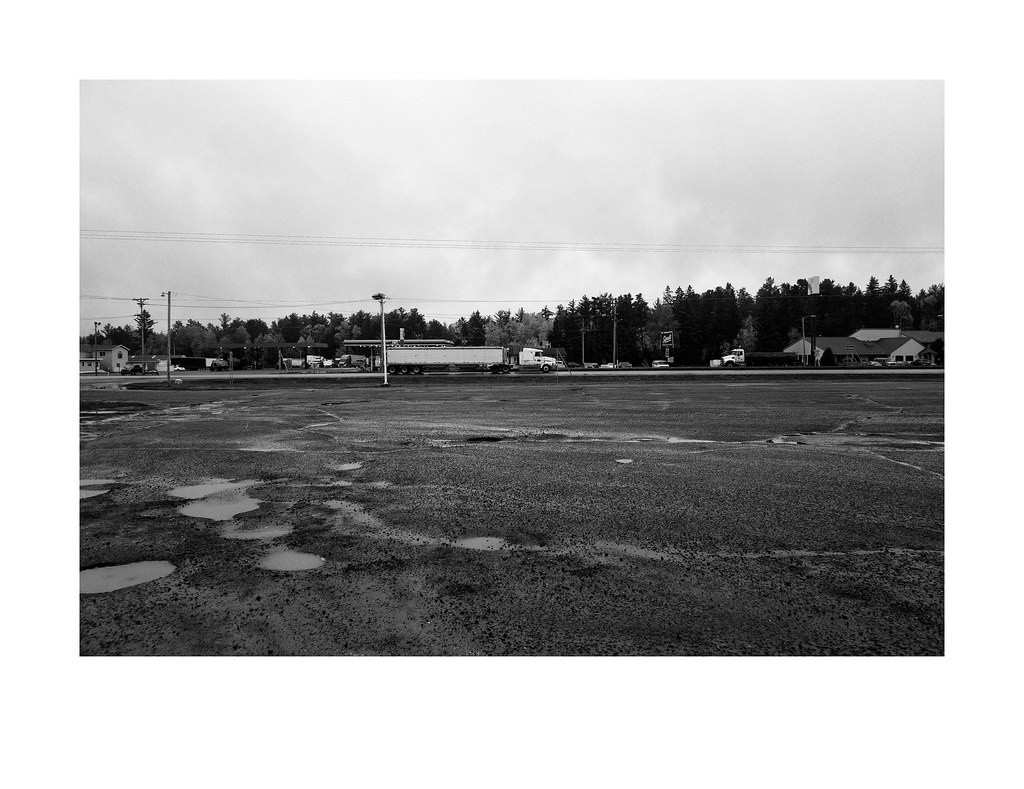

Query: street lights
[
  {"left": 161, "top": 292, "right": 171, "bottom": 386},
  {"left": 802, "top": 315, "right": 817, "bottom": 366},
  {"left": 94, "top": 321, "right": 101, "bottom": 375},
  {"left": 602, "top": 314, "right": 616, "bottom": 369},
  {"left": 371, "top": 293, "right": 388, "bottom": 385}
]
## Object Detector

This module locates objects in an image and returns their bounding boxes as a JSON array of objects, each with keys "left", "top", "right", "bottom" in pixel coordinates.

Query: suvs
[
  {"left": 652, "top": 360, "right": 670, "bottom": 368},
  {"left": 210, "top": 359, "right": 228, "bottom": 371}
]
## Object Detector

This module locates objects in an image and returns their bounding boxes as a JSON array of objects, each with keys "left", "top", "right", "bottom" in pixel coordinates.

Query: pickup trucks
[{"left": 121, "top": 364, "right": 143, "bottom": 376}]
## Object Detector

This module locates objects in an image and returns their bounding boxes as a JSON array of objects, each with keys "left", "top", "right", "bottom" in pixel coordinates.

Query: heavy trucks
[
  {"left": 338, "top": 354, "right": 366, "bottom": 368},
  {"left": 386, "top": 346, "right": 557, "bottom": 375},
  {"left": 276, "top": 358, "right": 302, "bottom": 369}
]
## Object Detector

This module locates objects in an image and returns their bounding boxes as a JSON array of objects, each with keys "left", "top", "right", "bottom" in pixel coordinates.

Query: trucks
[
  {"left": 601, "top": 363, "right": 616, "bottom": 369},
  {"left": 874, "top": 358, "right": 891, "bottom": 365},
  {"left": 721, "top": 349, "right": 797, "bottom": 368}
]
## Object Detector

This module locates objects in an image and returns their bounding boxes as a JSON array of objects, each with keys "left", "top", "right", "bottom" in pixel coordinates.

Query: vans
[
  {"left": 585, "top": 363, "right": 598, "bottom": 368},
  {"left": 886, "top": 362, "right": 897, "bottom": 366}
]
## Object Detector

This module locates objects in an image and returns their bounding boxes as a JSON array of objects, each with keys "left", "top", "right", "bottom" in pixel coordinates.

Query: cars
[
  {"left": 566, "top": 362, "right": 579, "bottom": 368},
  {"left": 616, "top": 362, "right": 633, "bottom": 368},
  {"left": 556, "top": 361, "right": 565, "bottom": 369},
  {"left": 174, "top": 365, "right": 185, "bottom": 371}
]
